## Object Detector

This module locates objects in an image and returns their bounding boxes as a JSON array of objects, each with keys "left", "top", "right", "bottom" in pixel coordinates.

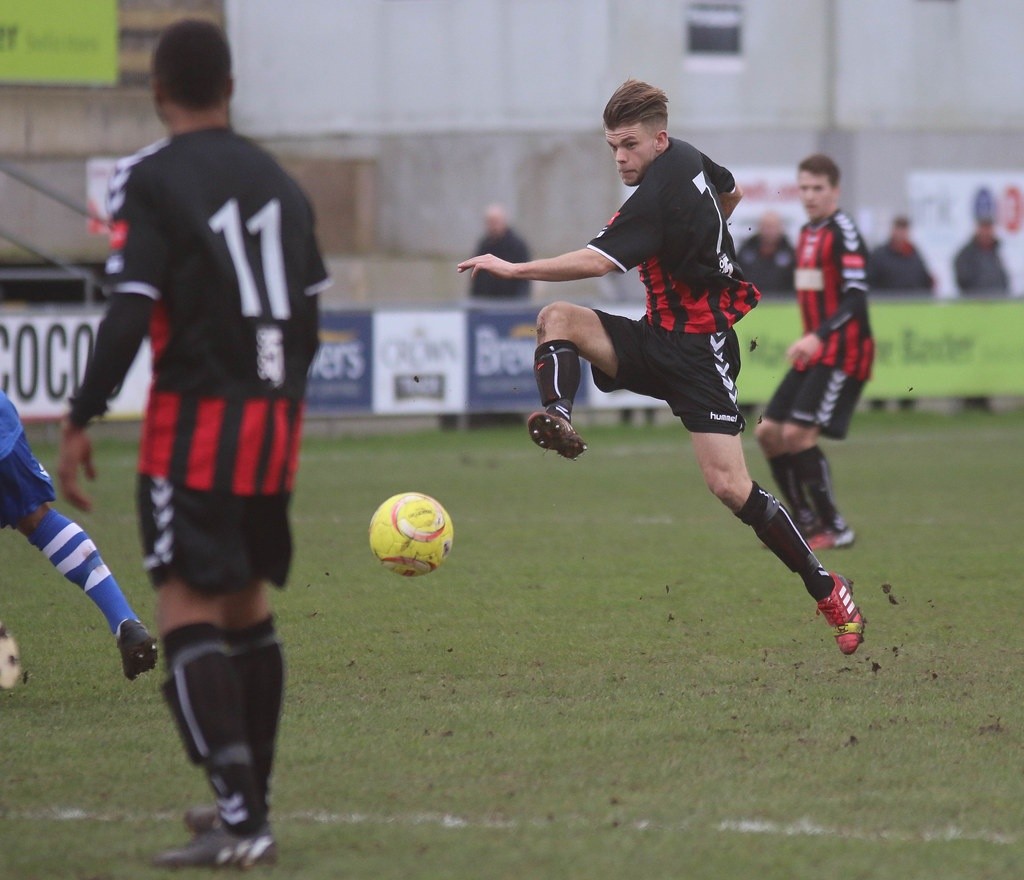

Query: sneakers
[
  {"left": 0, "top": 622, "right": 20, "bottom": 690},
  {"left": 528, "top": 413, "right": 586, "bottom": 460},
  {"left": 816, "top": 573, "right": 866, "bottom": 655},
  {"left": 186, "top": 801, "right": 224, "bottom": 834},
  {"left": 114, "top": 619, "right": 158, "bottom": 680},
  {"left": 154, "top": 819, "right": 277, "bottom": 867}
]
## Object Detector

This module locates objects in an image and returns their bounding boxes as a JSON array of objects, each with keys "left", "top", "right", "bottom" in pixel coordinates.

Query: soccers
[{"left": 367, "top": 492, "right": 454, "bottom": 578}]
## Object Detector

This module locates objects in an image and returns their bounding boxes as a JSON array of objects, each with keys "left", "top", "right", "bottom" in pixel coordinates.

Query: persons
[
  {"left": 457, "top": 79, "right": 864, "bottom": 652},
  {"left": 469, "top": 202, "right": 797, "bottom": 429},
  {"left": 54, "top": 16, "right": 328, "bottom": 868},
  {"left": 0, "top": 389, "right": 159, "bottom": 688},
  {"left": 750, "top": 152, "right": 876, "bottom": 549},
  {"left": 953, "top": 219, "right": 1007, "bottom": 411},
  {"left": 867, "top": 215, "right": 938, "bottom": 411}
]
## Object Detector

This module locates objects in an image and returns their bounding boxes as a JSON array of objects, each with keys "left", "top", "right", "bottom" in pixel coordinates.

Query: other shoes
[
  {"left": 825, "top": 525, "right": 854, "bottom": 547},
  {"left": 806, "top": 532, "right": 831, "bottom": 552}
]
